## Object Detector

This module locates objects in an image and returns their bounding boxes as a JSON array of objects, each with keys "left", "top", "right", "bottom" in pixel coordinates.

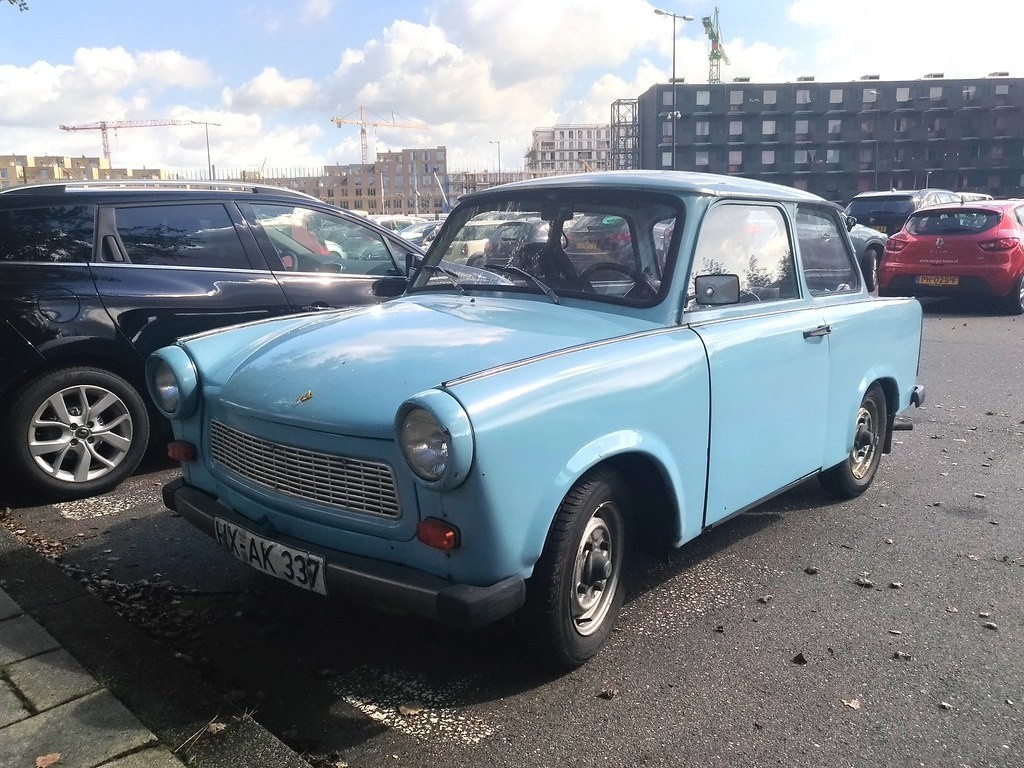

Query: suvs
[{"left": 1, "top": 182, "right": 521, "bottom": 501}]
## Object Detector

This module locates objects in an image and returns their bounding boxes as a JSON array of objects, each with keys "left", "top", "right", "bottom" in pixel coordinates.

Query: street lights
[{"left": 655, "top": 7, "right": 697, "bottom": 170}]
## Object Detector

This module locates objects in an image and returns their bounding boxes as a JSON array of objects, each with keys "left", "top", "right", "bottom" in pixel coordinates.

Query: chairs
[{"left": 504, "top": 241, "right": 581, "bottom": 294}]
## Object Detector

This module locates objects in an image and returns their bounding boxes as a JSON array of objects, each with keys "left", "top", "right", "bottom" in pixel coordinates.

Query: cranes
[
  {"left": 330, "top": 104, "right": 430, "bottom": 165},
  {"left": 59, "top": 117, "right": 195, "bottom": 169},
  {"left": 700, "top": 6, "right": 731, "bottom": 85}
]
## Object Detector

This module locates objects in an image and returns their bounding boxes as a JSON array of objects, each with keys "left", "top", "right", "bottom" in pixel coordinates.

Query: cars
[
  {"left": 145, "top": 167, "right": 924, "bottom": 668},
  {"left": 878, "top": 200, "right": 1023, "bottom": 316},
  {"left": 272, "top": 181, "right": 994, "bottom": 292}
]
